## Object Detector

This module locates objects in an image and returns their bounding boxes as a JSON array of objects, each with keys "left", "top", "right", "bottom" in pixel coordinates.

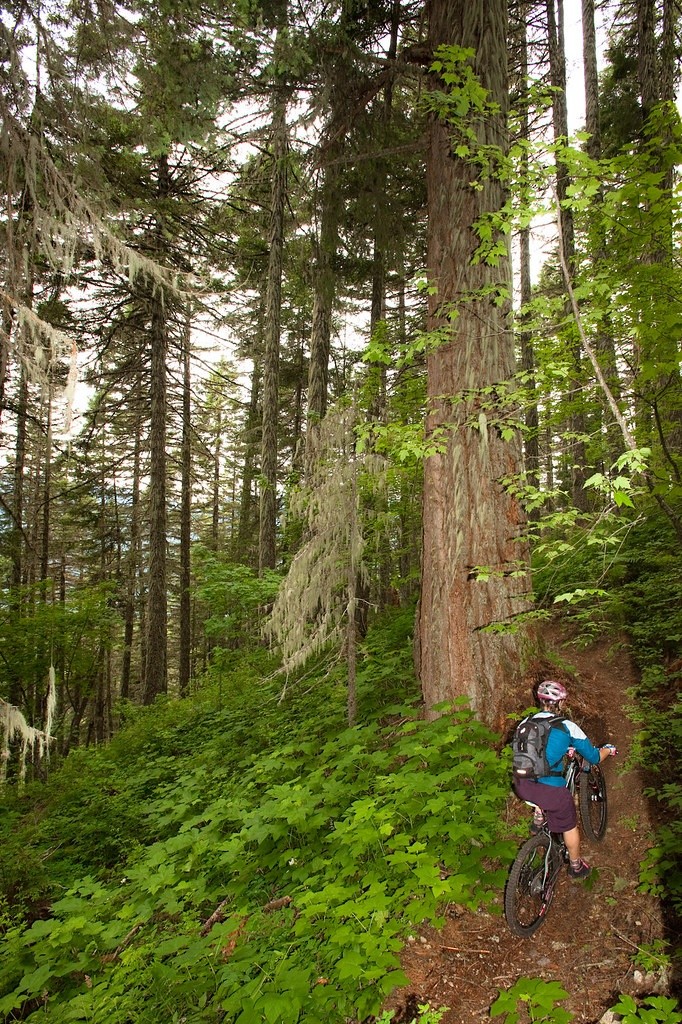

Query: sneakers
[
  {"left": 529, "top": 821, "right": 546, "bottom": 836},
  {"left": 567, "top": 861, "right": 592, "bottom": 881}
]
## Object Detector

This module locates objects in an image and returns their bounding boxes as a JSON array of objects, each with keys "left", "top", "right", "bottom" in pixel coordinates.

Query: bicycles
[{"left": 503, "top": 744, "right": 622, "bottom": 939}]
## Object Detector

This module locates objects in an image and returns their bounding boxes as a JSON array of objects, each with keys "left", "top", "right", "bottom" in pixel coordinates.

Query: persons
[{"left": 513, "top": 680, "right": 617, "bottom": 879}]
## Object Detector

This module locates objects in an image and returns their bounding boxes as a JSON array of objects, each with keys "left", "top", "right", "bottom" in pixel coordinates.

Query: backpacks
[{"left": 511, "top": 712, "right": 568, "bottom": 780}]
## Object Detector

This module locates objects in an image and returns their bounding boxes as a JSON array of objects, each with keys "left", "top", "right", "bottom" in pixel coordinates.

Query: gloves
[
  {"left": 565, "top": 746, "right": 576, "bottom": 758},
  {"left": 603, "top": 744, "right": 619, "bottom": 756}
]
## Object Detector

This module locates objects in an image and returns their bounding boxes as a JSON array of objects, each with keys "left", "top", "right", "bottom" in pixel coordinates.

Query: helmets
[{"left": 538, "top": 681, "right": 567, "bottom": 704}]
